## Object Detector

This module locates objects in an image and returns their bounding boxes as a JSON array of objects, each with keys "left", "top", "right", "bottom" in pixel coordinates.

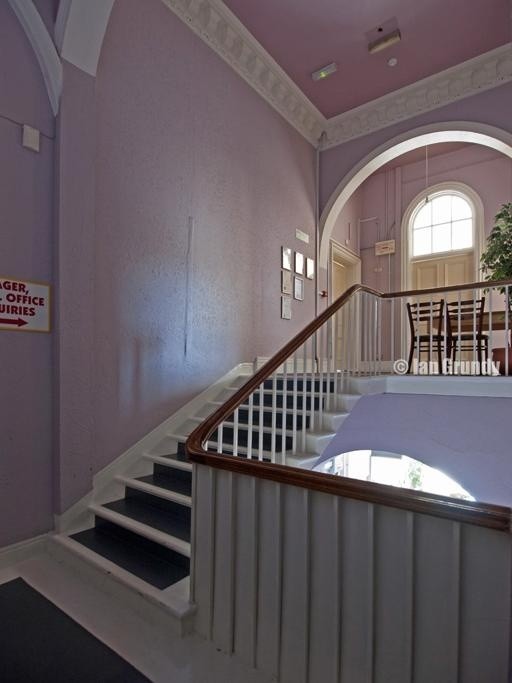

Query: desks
[{"left": 430, "top": 309, "right": 512, "bottom": 332}]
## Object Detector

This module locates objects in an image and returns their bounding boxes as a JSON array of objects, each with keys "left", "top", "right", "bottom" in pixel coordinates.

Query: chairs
[{"left": 405, "top": 296, "right": 489, "bottom": 374}]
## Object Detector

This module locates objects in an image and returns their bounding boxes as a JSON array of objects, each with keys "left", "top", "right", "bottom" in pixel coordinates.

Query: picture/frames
[{"left": 280, "top": 245, "right": 316, "bottom": 321}]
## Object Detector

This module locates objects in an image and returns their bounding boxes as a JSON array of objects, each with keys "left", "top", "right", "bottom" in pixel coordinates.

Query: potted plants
[{"left": 477, "top": 200, "right": 512, "bottom": 376}]
